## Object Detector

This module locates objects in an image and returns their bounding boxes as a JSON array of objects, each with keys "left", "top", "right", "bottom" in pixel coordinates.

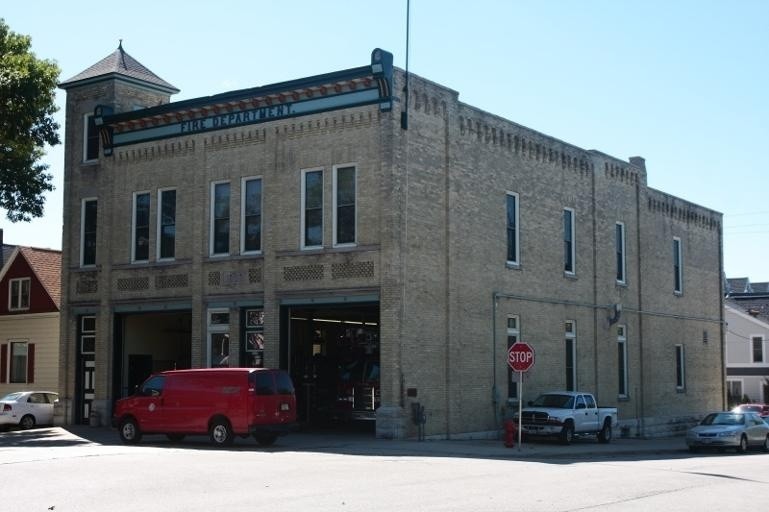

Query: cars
[
  {"left": 730, "top": 403, "right": 769, "bottom": 418},
  {"left": 686, "top": 412, "right": 768, "bottom": 451},
  {"left": 0, "top": 390, "right": 59, "bottom": 428}
]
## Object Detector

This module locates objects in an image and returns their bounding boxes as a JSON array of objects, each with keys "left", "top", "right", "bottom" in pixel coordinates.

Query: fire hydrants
[{"left": 505, "top": 417, "right": 516, "bottom": 447}]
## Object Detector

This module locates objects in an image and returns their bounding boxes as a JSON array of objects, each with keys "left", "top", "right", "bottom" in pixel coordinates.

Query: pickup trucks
[{"left": 514, "top": 389, "right": 619, "bottom": 445}]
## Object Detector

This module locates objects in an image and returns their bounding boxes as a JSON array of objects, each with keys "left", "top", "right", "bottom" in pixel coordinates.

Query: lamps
[{"left": 608, "top": 303, "right": 622, "bottom": 325}]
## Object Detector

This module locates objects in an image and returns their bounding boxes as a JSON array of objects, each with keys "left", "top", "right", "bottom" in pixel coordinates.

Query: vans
[{"left": 112, "top": 367, "right": 297, "bottom": 446}]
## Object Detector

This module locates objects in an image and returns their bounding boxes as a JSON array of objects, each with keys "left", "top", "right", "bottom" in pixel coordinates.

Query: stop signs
[{"left": 507, "top": 342, "right": 535, "bottom": 372}]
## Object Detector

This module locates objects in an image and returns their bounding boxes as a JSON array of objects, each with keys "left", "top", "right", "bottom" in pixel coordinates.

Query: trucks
[{"left": 332, "top": 338, "right": 381, "bottom": 423}]
